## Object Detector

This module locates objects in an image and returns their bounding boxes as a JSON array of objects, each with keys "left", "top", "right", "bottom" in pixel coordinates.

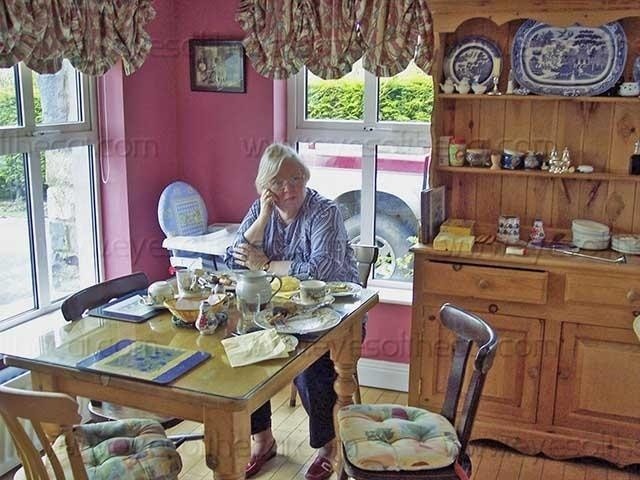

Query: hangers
[{"left": 137, "top": 294, "right": 152, "bottom": 306}]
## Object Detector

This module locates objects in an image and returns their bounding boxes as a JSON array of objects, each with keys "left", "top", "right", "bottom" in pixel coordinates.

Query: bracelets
[
  {"left": 162, "top": 291, "right": 228, "bottom": 326},
  {"left": 149, "top": 281, "right": 175, "bottom": 304}
]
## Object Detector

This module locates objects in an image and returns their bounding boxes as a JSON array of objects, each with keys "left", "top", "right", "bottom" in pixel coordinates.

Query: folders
[{"left": 0, "top": 360, "right": 95, "bottom": 476}]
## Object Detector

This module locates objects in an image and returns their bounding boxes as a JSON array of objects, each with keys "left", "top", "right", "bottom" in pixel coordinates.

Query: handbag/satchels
[{"left": 262, "top": 259, "right": 271, "bottom": 271}]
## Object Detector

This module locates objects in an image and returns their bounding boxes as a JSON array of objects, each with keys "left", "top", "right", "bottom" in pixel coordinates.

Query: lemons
[
  {"left": 231, "top": 269, "right": 282, "bottom": 315},
  {"left": 525, "top": 150, "right": 540, "bottom": 169}
]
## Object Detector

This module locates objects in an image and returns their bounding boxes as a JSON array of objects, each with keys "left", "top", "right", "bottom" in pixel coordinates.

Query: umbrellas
[
  {"left": 303, "top": 455, "right": 338, "bottom": 480},
  {"left": 245, "top": 439, "right": 278, "bottom": 479}
]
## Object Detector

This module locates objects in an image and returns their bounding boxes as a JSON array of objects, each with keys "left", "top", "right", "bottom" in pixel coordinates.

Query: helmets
[
  {"left": 571, "top": 219, "right": 640, "bottom": 255},
  {"left": 140, "top": 296, "right": 179, "bottom": 309},
  {"left": 254, "top": 282, "right": 362, "bottom": 352},
  {"left": 576, "top": 165, "right": 593, "bottom": 173},
  {"left": 439, "top": 17, "right": 640, "bottom": 96}
]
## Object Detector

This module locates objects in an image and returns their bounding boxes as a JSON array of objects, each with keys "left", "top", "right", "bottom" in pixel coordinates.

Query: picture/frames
[
  {"left": 0, "top": 278, "right": 381, "bottom": 480},
  {"left": 163, "top": 220, "right": 245, "bottom": 271}
]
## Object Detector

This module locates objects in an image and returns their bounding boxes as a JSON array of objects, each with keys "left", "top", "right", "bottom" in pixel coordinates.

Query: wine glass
[{"left": 266, "top": 190, "right": 270, "bottom": 197}]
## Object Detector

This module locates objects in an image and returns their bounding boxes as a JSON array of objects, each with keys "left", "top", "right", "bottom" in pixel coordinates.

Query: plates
[{"left": 402, "top": 1, "right": 640, "bottom": 471}]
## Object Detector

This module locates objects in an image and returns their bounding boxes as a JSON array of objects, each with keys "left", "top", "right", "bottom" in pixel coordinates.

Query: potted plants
[{"left": 187, "top": 37, "right": 248, "bottom": 95}]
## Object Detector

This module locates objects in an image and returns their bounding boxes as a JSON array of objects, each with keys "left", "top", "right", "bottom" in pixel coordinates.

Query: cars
[
  {"left": 176, "top": 269, "right": 197, "bottom": 296},
  {"left": 300, "top": 281, "right": 330, "bottom": 304},
  {"left": 467, "top": 149, "right": 491, "bottom": 167},
  {"left": 503, "top": 150, "right": 525, "bottom": 170}
]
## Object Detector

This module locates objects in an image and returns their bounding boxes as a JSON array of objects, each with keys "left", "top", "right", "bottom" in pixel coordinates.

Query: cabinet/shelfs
[
  {"left": 628, "top": 142, "right": 640, "bottom": 176},
  {"left": 491, "top": 151, "right": 502, "bottom": 170},
  {"left": 438, "top": 135, "right": 466, "bottom": 167},
  {"left": 496, "top": 215, "right": 520, "bottom": 244},
  {"left": 530, "top": 218, "right": 545, "bottom": 241},
  {"left": 196, "top": 272, "right": 231, "bottom": 334}
]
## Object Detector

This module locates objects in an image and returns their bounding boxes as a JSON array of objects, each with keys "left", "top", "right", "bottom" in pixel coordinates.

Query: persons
[{"left": 223, "top": 143, "right": 368, "bottom": 480}]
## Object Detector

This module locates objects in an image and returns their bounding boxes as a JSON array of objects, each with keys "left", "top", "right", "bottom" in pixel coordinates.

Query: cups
[{"left": 269, "top": 175, "right": 304, "bottom": 192}]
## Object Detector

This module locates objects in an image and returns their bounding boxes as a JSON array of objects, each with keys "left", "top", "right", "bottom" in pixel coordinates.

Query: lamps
[
  {"left": 158, "top": 180, "right": 219, "bottom": 274},
  {"left": 60, "top": 270, "right": 205, "bottom": 452},
  {"left": 287, "top": 242, "right": 379, "bottom": 409},
  {"left": 0, "top": 385, "right": 183, "bottom": 480},
  {"left": 330, "top": 302, "right": 500, "bottom": 480}
]
